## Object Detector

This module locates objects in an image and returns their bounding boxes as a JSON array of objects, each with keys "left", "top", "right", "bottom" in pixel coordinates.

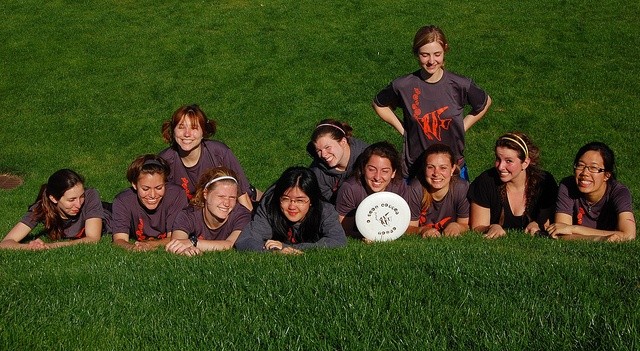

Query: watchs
[{"left": 191, "top": 235, "right": 199, "bottom": 246}]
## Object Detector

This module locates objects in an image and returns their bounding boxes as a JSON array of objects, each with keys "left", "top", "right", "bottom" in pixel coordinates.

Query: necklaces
[{"left": 579, "top": 182, "right": 607, "bottom": 212}]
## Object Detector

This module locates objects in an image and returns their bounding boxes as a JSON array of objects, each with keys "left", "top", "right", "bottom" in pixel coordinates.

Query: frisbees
[{"left": 355, "top": 192, "right": 411, "bottom": 243}]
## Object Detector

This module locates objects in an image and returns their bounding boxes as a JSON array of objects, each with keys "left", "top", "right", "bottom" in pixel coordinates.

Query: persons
[
  {"left": 373, "top": 25, "right": 492, "bottom": 181},
  {"left": 335, "top": 139, "right": 409, "bottom": 224},
  {"left": 158, "top": 103, "right": 264, "bottom": 212},
  {"left": 112, "top": 154, "right": 188, "bottom": 252},
  {"left": 0, "top": 168, "right": 112, "bottom": 250},
  {"left": 469, "top": 131, "right": 557, "bottom": 240},
  {"left": 165, "top": 166, "right": 252, "bottom": 258},
  {"left": 404, "top": 144, "right": 471, "bottom": 239},
  {"left": 546, "top": 141, "right": 637, "bottom": 244},
  {"left": 234, "top": 166, "right": 348, "bottom": 256},
  {"left": 308, "top": 118, "right": 371, "bottom": 204}
]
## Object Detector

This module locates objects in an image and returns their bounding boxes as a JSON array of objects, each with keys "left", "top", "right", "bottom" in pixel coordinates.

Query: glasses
[
  {"left": 279, "top": 196, "right": 310, "bottom": 206},
  {"left": 574, "top": 163, "right": 607, "bottom": 174}
]
